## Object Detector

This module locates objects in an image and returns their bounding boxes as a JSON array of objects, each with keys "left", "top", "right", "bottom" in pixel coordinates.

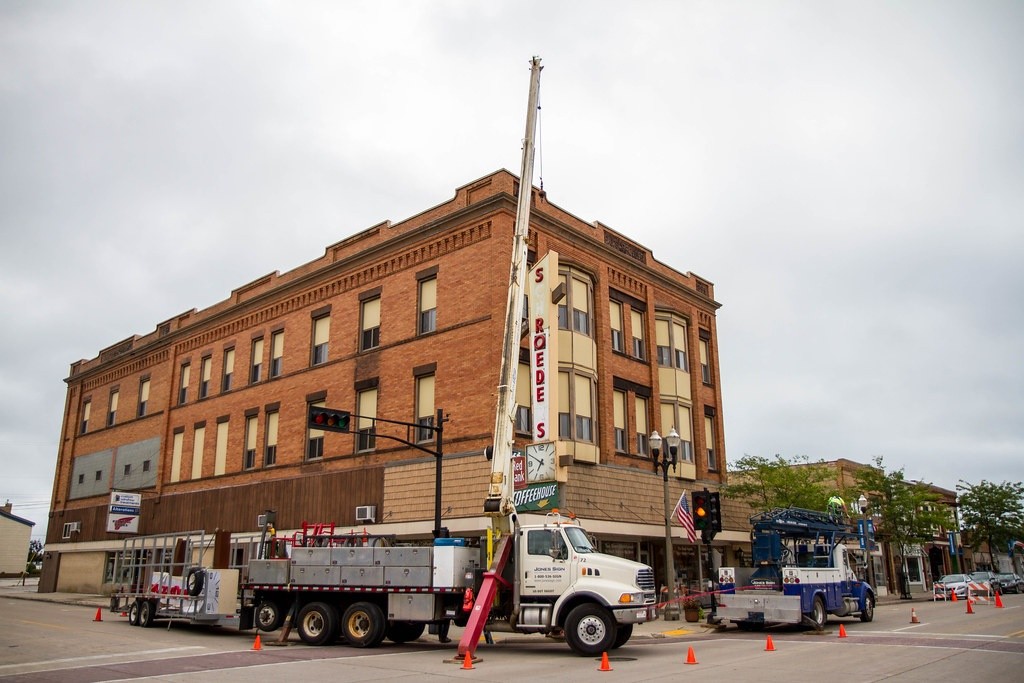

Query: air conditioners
[
  {"left": 355, "top": 506, "right": 375, "bottom": 520},
  {"left": 70, "top": 522, "right": 80, "bottom": 532},
  {"left": 257, "top": 515, "right": 271, "bottom": 527}
]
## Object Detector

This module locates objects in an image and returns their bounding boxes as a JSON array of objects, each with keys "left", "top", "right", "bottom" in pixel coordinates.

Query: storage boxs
[
  {"left": 387, "top": 594, "right": 445, "bottom": 621},
  {"left": 248, "top": 546, "right": 434, "bottom": 587}
]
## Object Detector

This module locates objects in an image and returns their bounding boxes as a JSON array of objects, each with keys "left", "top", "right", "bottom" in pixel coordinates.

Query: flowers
[{"left": 682, "top": 598, "right": 703, "bottom": 610}]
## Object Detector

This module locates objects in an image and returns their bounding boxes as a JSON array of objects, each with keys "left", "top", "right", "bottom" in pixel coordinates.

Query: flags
[{"left": 675, "top": 494, "right": 697, "bottom": 544}]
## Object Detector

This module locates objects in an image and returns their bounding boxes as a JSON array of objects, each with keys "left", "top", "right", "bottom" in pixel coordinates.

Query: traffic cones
[
  {"left": 909, "top": 608, "right": 920, "bottom": 623},
  {"left": 250, "top": 634, "right": 263, "bottom": 650},
  {"left": 763, "top": 634, "right": 777, "bottom": 651},
  {"left": 92, "top": 607, "right": 103, "bottom": 622},
  {"left": 596, "top": 652, "right": 614, "bottom": 671},
  {"left": 460, "top": 651, "right": 476, "bottom": 670},
  {"left": 837, "top": 623, "right": 848, "bottom": 638},
  {"left": 120, "top": 606, "right": 127, "bottom": 617},
  {"left": 994, "top": 591, "right": 1005, "bottom": 608},
  {"left": 683, "top": 645, "right": 699, "bottom": 665},
  {"left": 965, "top": 599, "right": 975, "bottom": 614},
  {"left": 951, "top": 590, "right": 958, "bottom": 601}
]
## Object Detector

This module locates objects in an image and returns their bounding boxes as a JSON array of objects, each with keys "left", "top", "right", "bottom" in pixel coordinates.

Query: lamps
[{"left": 735, "top": 547, "right": 744, "bottom": 561}]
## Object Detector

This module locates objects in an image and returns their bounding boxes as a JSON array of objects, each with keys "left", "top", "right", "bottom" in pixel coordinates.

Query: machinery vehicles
[
  {"left": 710, "top": 504, "right": 877, "bottom": 633},
  {"left": 243, "top": 53, "right": 655, "bottom": 666}
]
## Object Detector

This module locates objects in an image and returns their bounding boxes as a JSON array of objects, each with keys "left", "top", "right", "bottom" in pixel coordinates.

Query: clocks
[
  {"left": 195, "top": 569, "right": 239, "bottom": 615},
  {"left": 524, "top": 440, "right": 557, "bottom": 484}
]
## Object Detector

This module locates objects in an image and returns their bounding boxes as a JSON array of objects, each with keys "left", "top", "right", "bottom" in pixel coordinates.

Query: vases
[{"left": 684, "top": 609, "right": 699, "bottom": 622}]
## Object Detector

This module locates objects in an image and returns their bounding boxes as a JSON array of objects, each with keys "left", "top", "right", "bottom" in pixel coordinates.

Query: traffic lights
[
  {"left": 308, "top": 405, "right": 351, "bottom": 434},
  {"left": 691, "top": 490, "right": 712, "bottom": 530}
]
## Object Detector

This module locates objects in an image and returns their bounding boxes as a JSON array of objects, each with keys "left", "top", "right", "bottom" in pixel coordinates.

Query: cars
[
  {"left": 996, "top": 573, "right": 1024, "bottom": 595},
  {"left": 967, "top": 571, "right": 1003, "bottom": 597},
  {"left": 932, "top": 570, "right": 972, "bottom": 600}
]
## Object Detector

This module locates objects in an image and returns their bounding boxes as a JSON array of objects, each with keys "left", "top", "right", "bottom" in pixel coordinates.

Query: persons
[
  {"left": 827, "top": 495, "right": 850, "bottom": 522},
  {"left": 268, "top": 527, "right": 275, "bottom": 538}
]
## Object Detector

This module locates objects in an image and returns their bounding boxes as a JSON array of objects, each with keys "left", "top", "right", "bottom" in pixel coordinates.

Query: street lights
[
  {"left": 858, "top": 494, "right": 875, "bottom": 594},
  {"left": 648, "top": 425, "right": 682, "bottom": 622}
]
